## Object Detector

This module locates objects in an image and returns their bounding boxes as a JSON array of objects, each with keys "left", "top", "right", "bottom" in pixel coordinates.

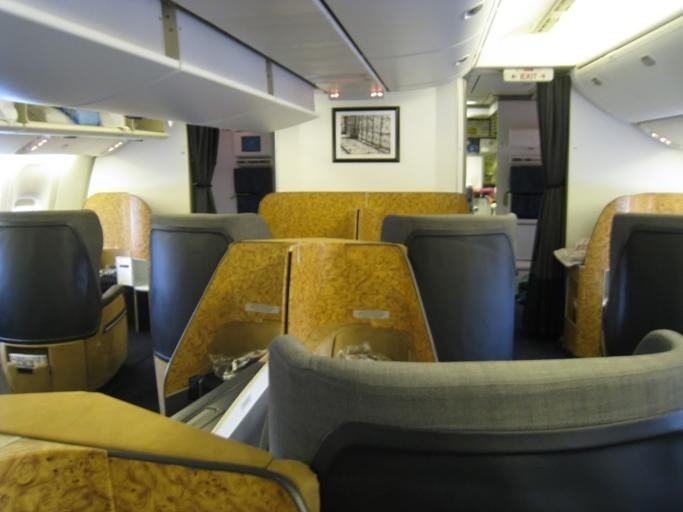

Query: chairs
[
  {"left": 160, "top": 237, "right": 439, "bottom": 433},
  {"left": 380, "top": 212, "right": 520, "bottom": 361},
  {"left": 0, "top": 207, "right": 130, "bottom": 396},
  {"left": 256, "top": 190, "right": 478, "bottom": 242},
  {"left": 265, "top": 328, "right": 683, "bottom": 512},
  {"left": 0, "top": 388, "right": 323, "bottom": 511},
  {"left": 561, "top": 191, "right": 683, "bottom": 358},
  {"left": 146, "top": 209, "right": 275, "bottom": 405},
  {"left": 81, "top": 191, "right": 151, "bottom": 336}
]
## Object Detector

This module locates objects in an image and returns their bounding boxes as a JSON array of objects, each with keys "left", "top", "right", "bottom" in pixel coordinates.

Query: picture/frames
[{"left": 331, "top": 106, "right": 400, "bottom": 163}]
